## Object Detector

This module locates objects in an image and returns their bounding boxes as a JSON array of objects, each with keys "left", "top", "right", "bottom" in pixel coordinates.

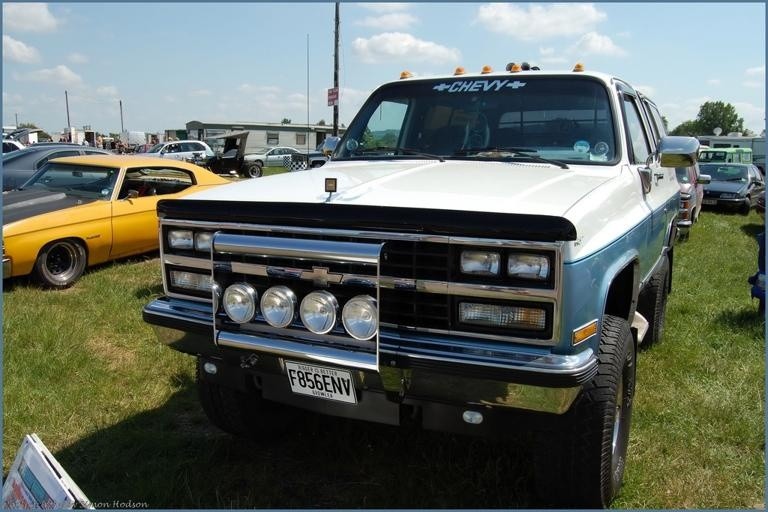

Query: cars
[{"left": 676, "top": 148, "right": 765, "bottom": 242}]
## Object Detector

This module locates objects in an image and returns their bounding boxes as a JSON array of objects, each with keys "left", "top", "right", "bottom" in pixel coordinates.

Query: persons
[
  {"left": 95, "top": 135, "right": 128, "bottom": 154},
  {"left": 150, "top": 135, "right": 182, "bottom": 153},
  {"left": 81, "top": 137, "right": 89, "bottom": 146}
]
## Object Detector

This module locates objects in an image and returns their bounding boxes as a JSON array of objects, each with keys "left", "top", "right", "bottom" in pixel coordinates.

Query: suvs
[{"left": 144, "top": 62, "right": 701, "bottom": 509}]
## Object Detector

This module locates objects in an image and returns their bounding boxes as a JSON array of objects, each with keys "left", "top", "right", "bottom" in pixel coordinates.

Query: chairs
[{"left": 436, "top": 126, "right": 467, "bottom": 150}]
